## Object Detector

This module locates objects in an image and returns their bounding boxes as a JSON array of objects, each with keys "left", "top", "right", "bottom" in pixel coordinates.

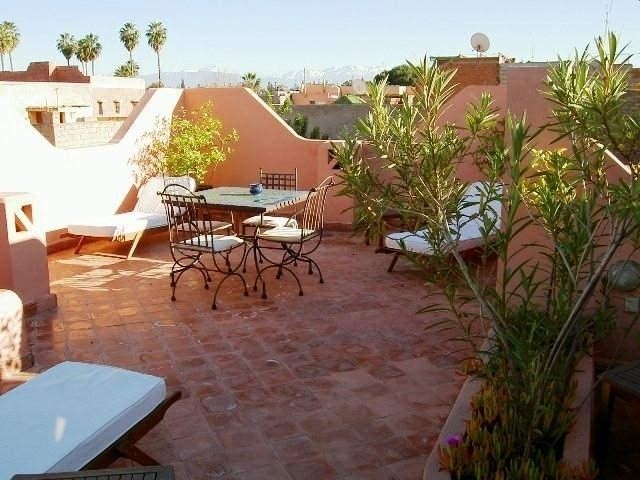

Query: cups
[{"left": 249, "top": 183, "right": 263, "bottom": 195}]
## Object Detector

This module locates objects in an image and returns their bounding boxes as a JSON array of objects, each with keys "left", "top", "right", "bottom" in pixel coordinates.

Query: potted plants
[{"left": 134, "top": 97, "right": 240, "bottom": 192}]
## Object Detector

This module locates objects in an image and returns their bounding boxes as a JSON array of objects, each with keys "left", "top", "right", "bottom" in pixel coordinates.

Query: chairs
[
  {"left": 68, "top": 168, "right": 335, "bottom": 310},
  {"left": 0, "top": 360, "right": 182, "bottom": 480},
  {"left": 375, "top": 180, "right": 503, "bottom": 278}
]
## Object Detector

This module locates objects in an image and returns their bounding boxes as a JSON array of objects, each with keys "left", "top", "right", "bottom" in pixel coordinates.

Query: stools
[{"left": 365, "top": 209, "right": 416, "bottom": 247}]
